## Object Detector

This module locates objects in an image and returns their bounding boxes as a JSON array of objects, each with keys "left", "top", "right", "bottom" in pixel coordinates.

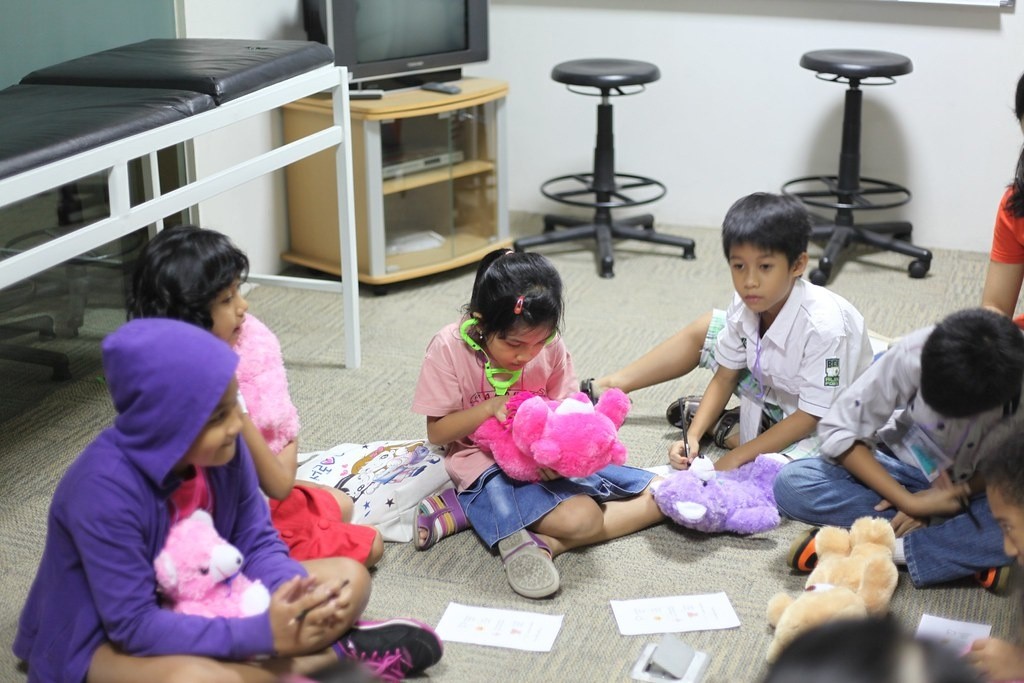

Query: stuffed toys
[
  {"left": 153, "top": 507, "right": 272, "bottom": 661},
  {"left": 649, "top": 452, "right": 790, "bottom": 535},
  {"left": 467, "top": 386, "right": 629, "bottom": 482},
  {"left": 232, "top": 311, "right": 301, "bottom": 457},
  {"left": 763, "top": 515, "right": 899, "bottom": 665}
]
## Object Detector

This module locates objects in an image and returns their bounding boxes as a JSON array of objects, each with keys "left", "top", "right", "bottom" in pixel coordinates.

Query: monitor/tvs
[{"left": 302, "top": 0, "right": 489, "bottom": 95}]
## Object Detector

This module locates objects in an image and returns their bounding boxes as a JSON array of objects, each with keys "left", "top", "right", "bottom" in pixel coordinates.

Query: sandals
[
  {"left": 413, "top": 488, "right": 466, "bottom": 551},
  {"left": 580, "top": 378, "right": 625, "bottom": 426},
  {"left": 667, "top": 397, "right": 741, "bottom": 449},
  {"left": 499, "top": 529, "right": 559, "bottom": 598}
]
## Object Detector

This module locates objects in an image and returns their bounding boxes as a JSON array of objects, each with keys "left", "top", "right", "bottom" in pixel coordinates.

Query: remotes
[
  {"left": 348, "top": 90, "right": 384, "bottom": 99},
  {"left": 422, "top": 81, "right": 461, "bottom": 95}
]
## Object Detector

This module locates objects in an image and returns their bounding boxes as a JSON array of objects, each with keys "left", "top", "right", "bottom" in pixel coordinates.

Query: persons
[
  {"left": 963, "top": 404, "right": 1024, "bottom": 683},
  {"left": 578, "top": 191, "right": 875, "bottom": 470},
  {"left": 12, "top": 317, "right": 445, "bottom": 683},
  {"left": 124, "top": 221, "right": 385, "bottom": 569},
  {"left": 772, "top": 68, "right": 1024, "bottom": 593},
  {"left": 412, "top": 248, "right": 668, "bottom": 596},
  {"left": 762, "top": 616, "right": 987, "bottom": 683}
]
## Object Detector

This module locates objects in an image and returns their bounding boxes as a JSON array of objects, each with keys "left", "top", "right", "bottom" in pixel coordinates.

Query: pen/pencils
[
  {"left": 948, "top": 467, "right": 985, "bottom": 534},
  {"left": 286, "top": 579, "right": 350, "bottom": 627},
  {"left": 678, "top": 398, "right": 692, "bottom": 468}
]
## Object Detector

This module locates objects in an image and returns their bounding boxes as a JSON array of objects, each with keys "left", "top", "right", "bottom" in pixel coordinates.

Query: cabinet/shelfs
[{"left": 275, "top": 75, "right": 514, "bottom": 295}]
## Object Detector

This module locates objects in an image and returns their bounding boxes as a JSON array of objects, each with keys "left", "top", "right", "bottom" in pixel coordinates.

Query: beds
[{"left": 0, "top": 37, "right": 359, "bottom": 370}]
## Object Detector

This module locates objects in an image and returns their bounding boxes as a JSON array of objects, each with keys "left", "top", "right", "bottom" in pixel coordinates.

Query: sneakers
[
  {"left": 331, "top": 618, "right": 443, "bottom": 683},
  {"left": 974, "top": 564, "right": 1010, "bottom": 593},
  {"left": 785, "top": 527, "right": 818, "bottom": 571}
]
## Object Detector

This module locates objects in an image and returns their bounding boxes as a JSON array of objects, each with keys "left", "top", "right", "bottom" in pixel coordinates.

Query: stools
[
  {"left": 512, "top": 57, "right": 695, "bottom": 280},
  {"left": 779, "top": 47, "right": 932, "bottom": 286}
]
